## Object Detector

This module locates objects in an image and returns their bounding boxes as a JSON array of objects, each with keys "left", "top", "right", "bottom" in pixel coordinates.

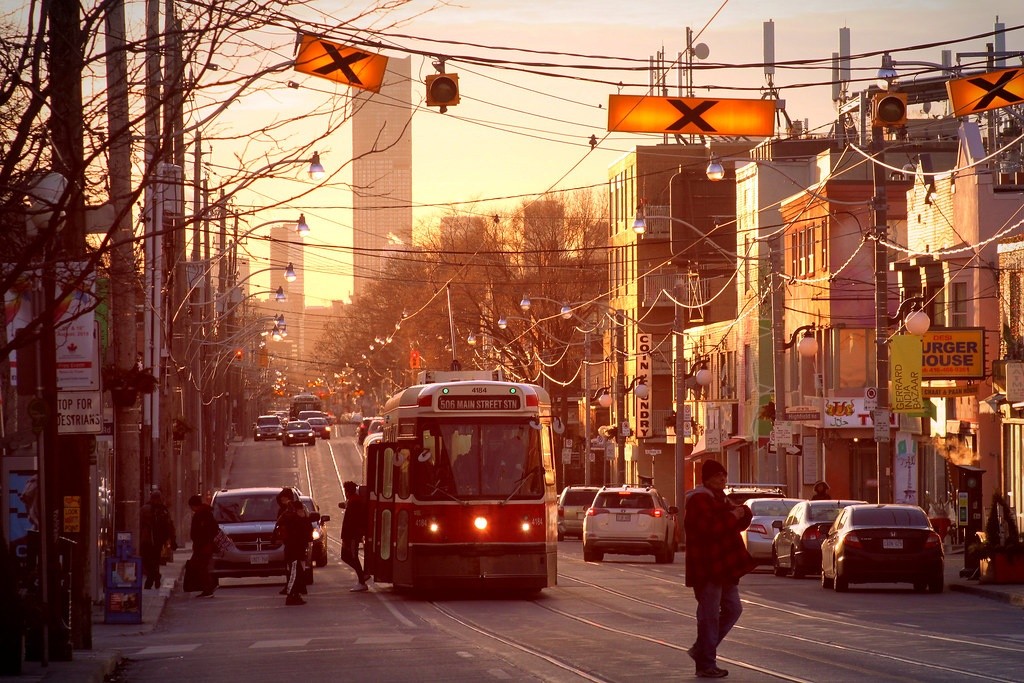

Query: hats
[{"left": 701, "top": 459, "right": 728, "bottom": 480}]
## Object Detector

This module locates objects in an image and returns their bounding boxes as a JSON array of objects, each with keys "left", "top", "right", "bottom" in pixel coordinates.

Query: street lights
[
  {"left": 495, "top": 311, "right": 616, "bottom": 489},
  {"left": 631, "top": 210, "right": 818, "bottom": 494},
  {"left": 559, "top": 282, "right": 715, "bottom": 545},
  {"left": 467, "top": 329, "right": 543, "bottom": 386},
  {"left": 707, "top": 84, "right": 932, "bottom": 504},
  {"left": 161, "top": 17, "right": 329, "bottom": 548},
  {"left": 519, "top": 293, "right": 650, "bottom": 487}
]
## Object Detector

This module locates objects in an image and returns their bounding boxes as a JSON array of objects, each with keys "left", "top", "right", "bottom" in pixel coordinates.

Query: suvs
[
  {"left": 205, "top": 486, "right": 322, "bottom": 586},
  {"left": 722, "top": 482, "right": 789, "bottom": 504},
  {"left": 558, "top": 483, "right": 601, "bottom": 542},
  {"left": 252, "top": 415, "right": 285, "bottom": 442},
  {"left": 581, "top": 484, "right": 679, "bottom": 564},
  {"left": 296, "top": 410, "right": 324, "bottom": 421}
]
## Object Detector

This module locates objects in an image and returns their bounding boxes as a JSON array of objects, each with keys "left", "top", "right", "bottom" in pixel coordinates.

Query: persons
[
  {"left": 458, "top": 445, "right": 482, "bottom": 464},
  {"left": 188, "top": 495, "right": 220, "bottom": 598},
  {"left": 271, "top": 487, "right": 308, "bottom": 595},
  {"left": 139, "top": 490, "right": 177, "bottom": 589},
  {"left": 811, "top": 481, "right": 832, "bottom": 500},
  {"left": 272, "top": 500, "right": 312, "bottom": 605},
  {"left": 341, "top": 481, "right": 371, "bottom": 591},
  {"left": 685, "top": 461, "right": 753, "bottom": 677}
]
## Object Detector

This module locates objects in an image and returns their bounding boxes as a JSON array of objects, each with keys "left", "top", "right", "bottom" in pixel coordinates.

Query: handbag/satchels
[{"left": 211, "top": 528, "right": 236, "bottom": 557}]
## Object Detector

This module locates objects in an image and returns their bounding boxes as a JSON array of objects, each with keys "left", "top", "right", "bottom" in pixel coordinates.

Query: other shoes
[
  {"left": 686, "top": 647, "right": 698, "bottom": 662},
  {"left": 155, "top": 573, "right": 162, "bottom": 589},
  {"left": 350, "top": 583, "right": 368, "bottom": 591},
  {"left": 694, "top": 665, "right": 729, "bottom": 678},
  {"left": 197, "top": 591, "right": 214, "bottom": 598},
  {"left": 286, "top": 596, "right": 306, "bottom": 605},
  {"left": 362, "top": 573, "right": 371, "bottom": 582},
  {"left": 301, "top": 589, "right": 308, "bottom": 595},
  {"left": 211, "top": 583, "right": 219, "bottom": 593},
  {"left": 144, "top": 576, "right": 154, "bottom": 589},
  {"left": 279, "top": 587, "right": 287, "bottom": 595}
]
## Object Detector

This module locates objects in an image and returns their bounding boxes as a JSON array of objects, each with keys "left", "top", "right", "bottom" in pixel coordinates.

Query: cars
[
  {"left": 737, "top": 498, "right": 809, "bottom": 563},
  {"left": 298, "top": 495, "right": 331, "bottom": 567},
  {"left": 280, "top": 420, "right": 316, "bottom": 447},
  {"left": 305, "top": 417, "right": 333, "bottom": 440},
  {"left": 770, "top": 498, "right": 870, "bottom": 579},
  {"left": 816, "top": 502, "right": 947, "bottom": 596}
]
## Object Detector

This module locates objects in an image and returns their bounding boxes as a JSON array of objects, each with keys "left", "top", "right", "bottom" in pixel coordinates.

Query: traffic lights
[
  {"left": 424, "top": 73, "right": 461, "bottom": 108},
  {"left": 873, "top": 92, "right": 909, "bottom": 126},
  {"left": 408, "top": 351, "right": 420, "bottom": 370}
]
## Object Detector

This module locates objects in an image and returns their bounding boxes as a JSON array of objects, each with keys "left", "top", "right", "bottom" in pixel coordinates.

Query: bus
[
  {"left": 358, "top": 282, "right": 565, "bottom": 596},
  {"left": 286, "top": 394, "right": 323, "bottom": 420}
]
